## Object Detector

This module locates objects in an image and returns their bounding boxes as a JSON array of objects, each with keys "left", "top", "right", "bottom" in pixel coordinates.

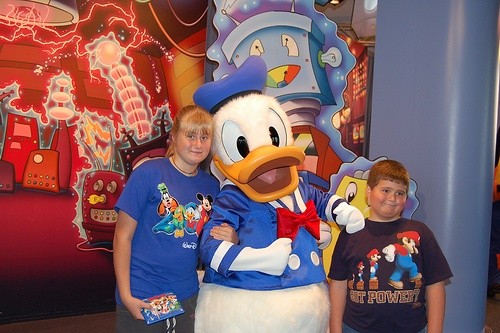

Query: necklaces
[{"left": 172, "top": 154, "right": 196, "bottom": 174}]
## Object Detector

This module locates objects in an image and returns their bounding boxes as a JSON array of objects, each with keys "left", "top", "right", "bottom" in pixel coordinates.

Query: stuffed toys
[{"left": 192, "top": 55, "right": 365, "bottom": 333}]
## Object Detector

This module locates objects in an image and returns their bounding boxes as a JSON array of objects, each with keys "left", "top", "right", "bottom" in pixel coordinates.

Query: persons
[
  {"left": 113, "top": 106, "right": 240, "bottom": 333},
  {"left": 328, "top": 160, "right": 453, "bottom": 333}
]
